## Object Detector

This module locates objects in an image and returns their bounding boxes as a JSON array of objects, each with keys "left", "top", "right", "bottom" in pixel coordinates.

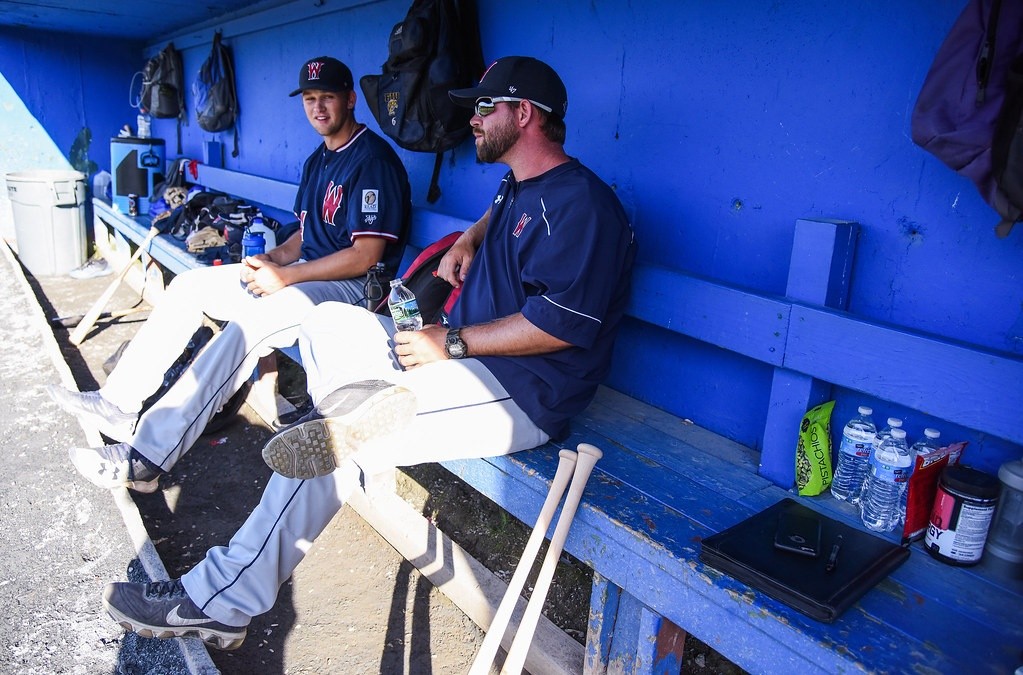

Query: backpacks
[
  {"left": 192, "top": 33, "right": 240, "bottom": 133},
  {"left": 911, "top": 0, "right": 1023, "bottom": 241},
  {"left": 359, "top": 0, "right": 489, "bottom": 155},
  {"left": 373, "top": 231, "right": 475, "bottom": 327},
  {"left": 141, "top": 44, "right": 186, "bottom": 119}
]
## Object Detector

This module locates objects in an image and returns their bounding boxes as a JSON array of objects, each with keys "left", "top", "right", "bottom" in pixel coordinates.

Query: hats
[
  {"left": 288, "top": 56, "right": 354, "bottom": 97},
  {"left": 448, "top": 56, "right": 568, "bottom": 119}
]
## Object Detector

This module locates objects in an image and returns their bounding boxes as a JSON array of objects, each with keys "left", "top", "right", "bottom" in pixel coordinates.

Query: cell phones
[{"left": 776, "top": 512, "right": 822, "bottom": 556}]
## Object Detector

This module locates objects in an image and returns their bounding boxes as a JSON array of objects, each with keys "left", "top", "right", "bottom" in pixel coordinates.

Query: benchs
[{"left": 91, "top": 160, "right": 1023, "bottom": 675}]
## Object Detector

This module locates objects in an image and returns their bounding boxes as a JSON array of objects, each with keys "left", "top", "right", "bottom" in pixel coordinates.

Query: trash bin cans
[{"left": 7, "top": 170, "right": 89, "bottom": 277}]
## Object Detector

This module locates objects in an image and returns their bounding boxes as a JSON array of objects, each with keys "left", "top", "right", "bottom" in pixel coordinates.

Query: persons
[
  {"left": 47, "top": 55, "right": 411, "bottom": 492},
  {"left": 103, "top": 55, "right": 631, "bottom": 651}
]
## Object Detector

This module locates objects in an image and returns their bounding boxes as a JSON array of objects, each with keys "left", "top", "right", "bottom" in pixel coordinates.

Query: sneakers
[
  {"left": 100, "top": 577, "right": 247, "bottom": 651},
  {"left": 261, "top": 380, "right": 418, "bottom": 480},
  {"left": 46, "top": 383, "right": 140, "bottom": 443},
  {"left": 68, "top": 442, "right": 165, "bottom": 493},
  {"left": 70, "top": 258, "right": 114, "bottom": 279}
]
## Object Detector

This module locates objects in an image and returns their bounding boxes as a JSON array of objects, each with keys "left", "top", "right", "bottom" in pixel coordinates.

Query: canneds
[{"left": 128, "top": 194, "right": 138, "bottom": 216}]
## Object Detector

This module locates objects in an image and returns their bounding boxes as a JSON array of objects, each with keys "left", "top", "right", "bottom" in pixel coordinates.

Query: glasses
[{"left": 474, "top": 96, "right": 552, "bottom": 116}]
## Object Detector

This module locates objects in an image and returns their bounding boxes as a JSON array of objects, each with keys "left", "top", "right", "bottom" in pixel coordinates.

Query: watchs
[{"left": 445, "top": 328, "right": 468, "bottom": 359}]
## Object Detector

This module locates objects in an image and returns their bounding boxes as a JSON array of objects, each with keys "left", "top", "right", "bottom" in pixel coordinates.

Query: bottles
[
  {"left": 899, "top": 427, "right": 941, "bottom": 527},
  {"left": 984, "top": 463, "right": 1023, "bottom": 565},
  {"left": 93, "top": 169, "right": 111, "bottom": 199},
  {"left": 241, "top": 217, "right": 276, "bottom": 258},
  {"left": 831, "top": 406, "right": 876, "bottom": 501},
  {"left": 129, "top": 192, "right": 137, "bottom": 217},
  {"left": 137, "top": 108, "right": 151, "bottom": 138},
  {"left": 243, "top": 231, "right": 266, "bottom": 257},
  {"left": 857, "top": 417, "right": 903, "bottom": 509},
  {"left": 388, "top": 279, "right": 422, "bottom": 331},
  {"left": 861, "top": 429, "right": 911, "bottom": 532}
]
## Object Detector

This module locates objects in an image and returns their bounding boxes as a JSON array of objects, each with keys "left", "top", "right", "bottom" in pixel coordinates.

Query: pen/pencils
[{"left": 824, "top": 532, "right": 844, "bottom": 572}]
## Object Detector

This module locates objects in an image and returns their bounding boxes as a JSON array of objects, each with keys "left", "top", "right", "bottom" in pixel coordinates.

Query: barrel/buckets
[
  {"left": 924, "top": 467, "right": 1004, "bottom": 568},
  {"left": 5, "top": 170, "right": 88, "bottom": 275}
]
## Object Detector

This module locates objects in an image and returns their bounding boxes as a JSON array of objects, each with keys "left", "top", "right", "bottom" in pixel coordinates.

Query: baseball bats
[
  {"left": 498, "top": 443, "right": 603, "bottom": 675},
  {"left": 49, "top": 305, "right": 152, "bottom": 330},
  {"left": 66, "top": 226, "right": 159, "bottom": 348},
  {"left": 466, "top": 449, "right": 577, "bottom": 675}
]
